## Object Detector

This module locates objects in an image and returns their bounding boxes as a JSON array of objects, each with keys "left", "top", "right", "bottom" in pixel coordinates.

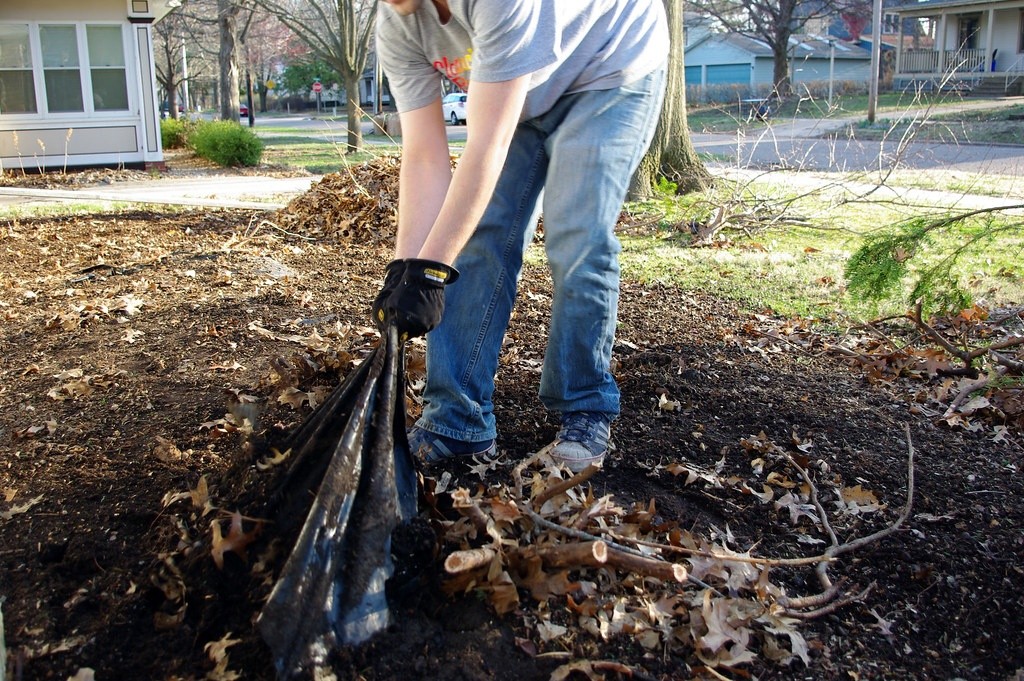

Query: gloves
[{"left": 372, "top": 258, "right": 461, "bottom": 346}]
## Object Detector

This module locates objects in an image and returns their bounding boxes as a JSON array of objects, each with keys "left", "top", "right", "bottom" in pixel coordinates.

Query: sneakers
[
  {"left": 406, "top": 425, "right": 496, "bottom": 466},
  {"left": 547, "top": 412, "right": 611, "bottom": 473}
]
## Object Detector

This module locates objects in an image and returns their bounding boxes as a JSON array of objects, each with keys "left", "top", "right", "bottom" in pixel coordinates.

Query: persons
[{"left": 371, "top": 0, "right": 669, "bottom": 475}]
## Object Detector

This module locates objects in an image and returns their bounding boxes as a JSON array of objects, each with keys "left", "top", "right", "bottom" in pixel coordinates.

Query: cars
[
  {"left": 160, "top": 102, "right": 183, "bottom": 112},
  {"left": 240, "top": 104, "right": 248, "bottom": 117},
  {"left": 443, "top": 93, "right": 467, "bottom": 125}
]
